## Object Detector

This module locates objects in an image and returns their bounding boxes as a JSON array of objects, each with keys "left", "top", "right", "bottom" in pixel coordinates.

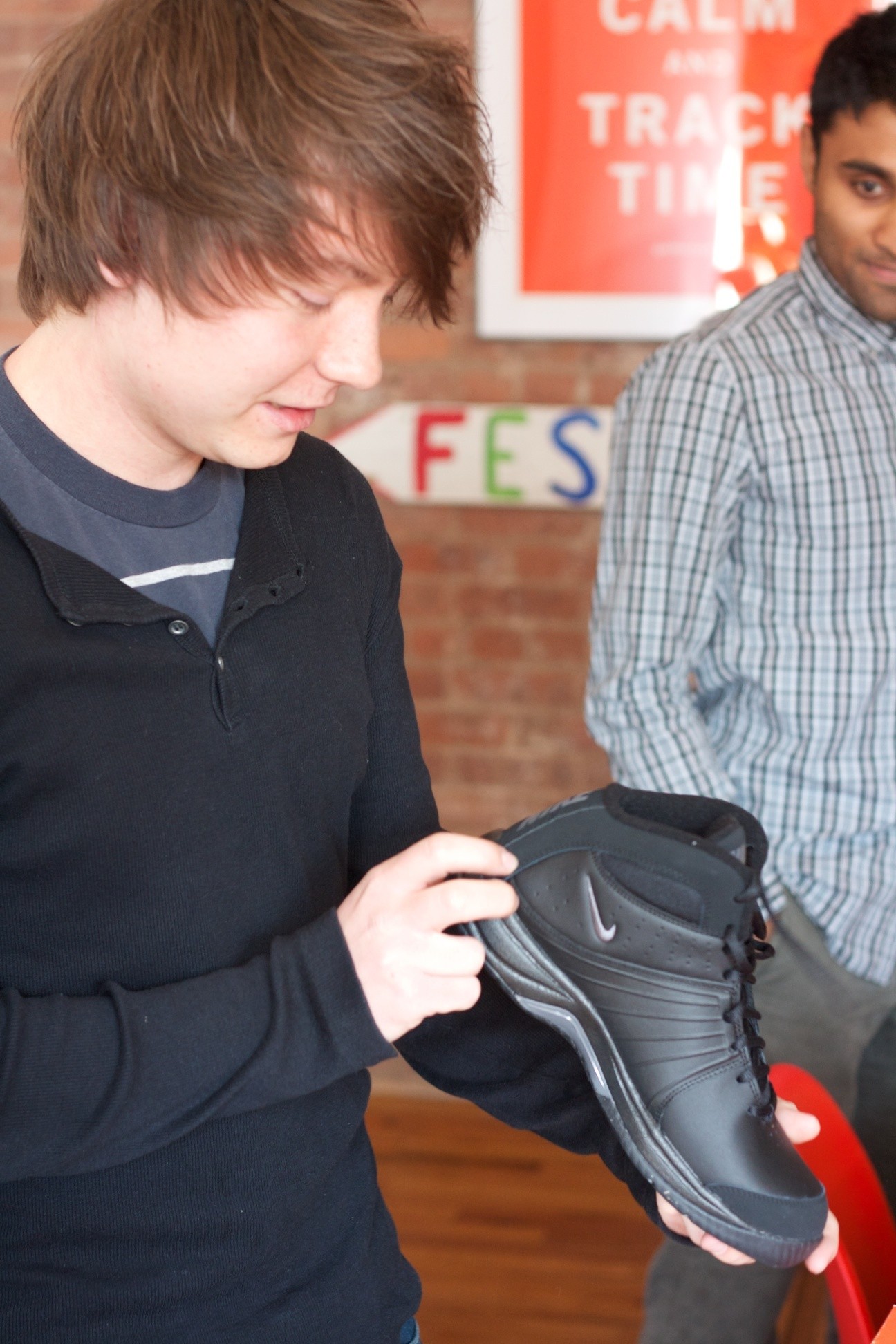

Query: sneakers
[{"left": 455, "top": 782, "right": 829, "bottom": 1268}]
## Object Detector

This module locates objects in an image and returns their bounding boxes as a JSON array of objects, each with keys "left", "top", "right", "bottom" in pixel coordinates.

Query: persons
[
  {"left": 0, "top": 1, "right": 840, "bottom": 1344},
  {"left": 582, "top": 4, "right": 895, "bottom": 1344}
]
